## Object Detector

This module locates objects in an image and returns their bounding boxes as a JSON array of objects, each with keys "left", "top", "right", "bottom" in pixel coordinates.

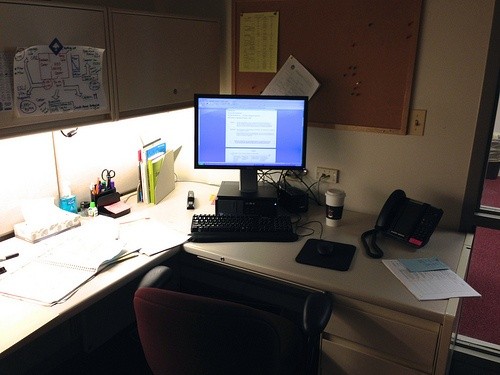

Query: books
[
  {"left": 1, "top": 218, "right": 192, "bottom": 305},
  {"left": 137, "top": 137, "right": 175, "bottom": 204}
]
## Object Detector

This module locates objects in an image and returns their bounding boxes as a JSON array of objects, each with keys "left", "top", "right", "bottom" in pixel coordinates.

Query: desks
[{"left": 0, "top": 181, "right": 474, "bottom": 375}]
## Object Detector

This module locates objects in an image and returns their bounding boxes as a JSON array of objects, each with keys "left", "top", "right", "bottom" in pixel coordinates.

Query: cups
[{"left": 325, "top": 189, "right": 347, "bottom": 227}]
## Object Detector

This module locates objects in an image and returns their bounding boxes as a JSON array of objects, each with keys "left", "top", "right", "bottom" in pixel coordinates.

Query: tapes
[{"left": 81, "top": 201, "right": 90, "bottom": 208}]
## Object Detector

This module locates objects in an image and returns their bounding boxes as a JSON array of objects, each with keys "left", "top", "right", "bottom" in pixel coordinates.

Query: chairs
[{"left": 134, "top": 263, "right": 333, "bottom": 375}]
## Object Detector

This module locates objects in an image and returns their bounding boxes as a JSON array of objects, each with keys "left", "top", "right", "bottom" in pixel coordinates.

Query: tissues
[{"left": 13, "top": 200, "right": 82, "bottom": 245}]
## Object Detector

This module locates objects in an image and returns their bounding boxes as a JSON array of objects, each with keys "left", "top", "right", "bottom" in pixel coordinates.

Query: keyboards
[{"left": 192, "top": 215, "right": 299, "bottom": 243}]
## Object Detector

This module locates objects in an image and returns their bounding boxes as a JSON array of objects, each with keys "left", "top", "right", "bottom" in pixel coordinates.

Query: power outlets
[{"left": 317, "top": 167, "right": 338, "bottom": 184}]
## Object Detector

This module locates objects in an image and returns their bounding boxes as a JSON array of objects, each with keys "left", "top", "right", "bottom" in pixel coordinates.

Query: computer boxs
[{"left": 214, "top": 180, "right": 282, "bottom": 216}]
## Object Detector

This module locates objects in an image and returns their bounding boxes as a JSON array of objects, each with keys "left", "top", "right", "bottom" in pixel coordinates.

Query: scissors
[{"left": 101, "top": 169, "right": 115, "bottom": 188}]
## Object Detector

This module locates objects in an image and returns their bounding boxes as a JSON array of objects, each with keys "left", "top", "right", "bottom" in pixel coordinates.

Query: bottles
[
  {"left": 80, "top": 201, "right": 90, "bottom": 217},
  {"left": 88, "top": 202, "right": 98, "bottom": 217}
]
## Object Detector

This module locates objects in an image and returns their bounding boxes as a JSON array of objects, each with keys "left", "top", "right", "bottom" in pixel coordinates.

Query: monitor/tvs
[{"left": 193, "top": 92, "right": 309, "bottom": 199}]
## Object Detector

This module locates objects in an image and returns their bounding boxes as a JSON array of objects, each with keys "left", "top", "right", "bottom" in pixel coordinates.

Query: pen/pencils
[
  {"left": 94, "top": 181, "right": 114, "bottom": 195},
  {"left": 0, "top": 252, "right": 20, "bottom": 262}
]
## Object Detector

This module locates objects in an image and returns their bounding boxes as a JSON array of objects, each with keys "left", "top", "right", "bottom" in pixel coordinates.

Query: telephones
[{"left": 374, "top": 188, "right": 444, "bottom": 248}]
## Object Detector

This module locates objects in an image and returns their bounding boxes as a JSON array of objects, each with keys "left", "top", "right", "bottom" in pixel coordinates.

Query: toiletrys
[{"left": 59, "top": 186, "right": 78, "bottom": 214}]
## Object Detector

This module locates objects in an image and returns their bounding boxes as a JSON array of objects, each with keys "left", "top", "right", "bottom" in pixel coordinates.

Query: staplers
[{"left": 186, "top": 191, "right": 194, "bottom": 210}]
